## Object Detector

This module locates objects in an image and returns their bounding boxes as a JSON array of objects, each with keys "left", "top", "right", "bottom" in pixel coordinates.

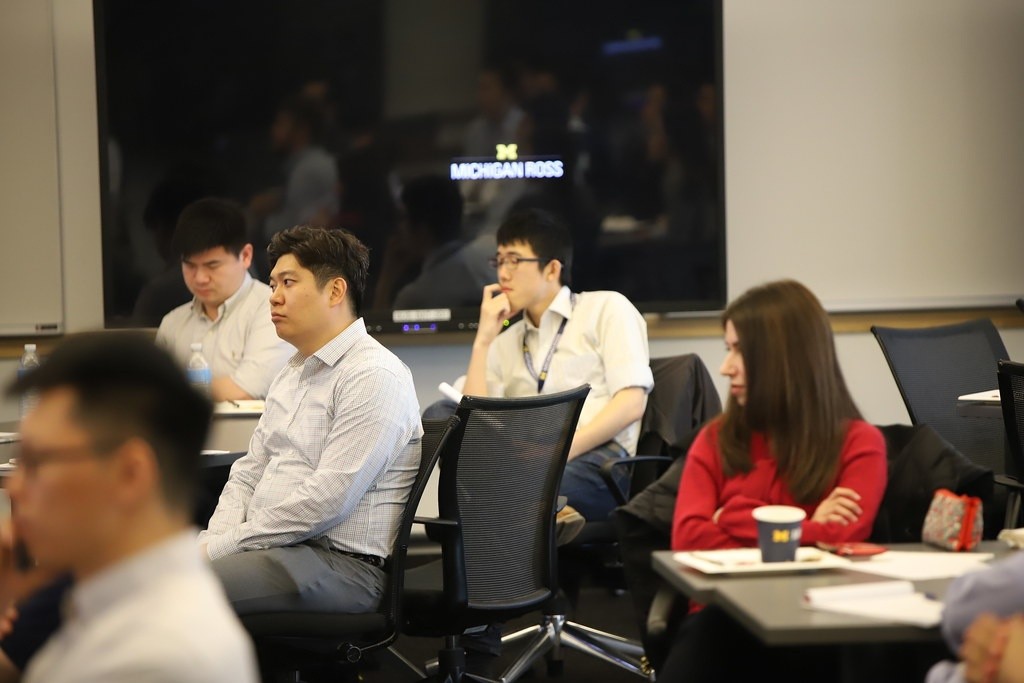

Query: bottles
[
  {"left": 187, "top": 343, "right": 211, "bottom": 395},
  {"left": 18, "top": 343, "right": 40, "bottom": 421}
]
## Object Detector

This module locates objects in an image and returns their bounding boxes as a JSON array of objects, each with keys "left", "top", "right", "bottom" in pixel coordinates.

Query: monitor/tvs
[{"left": 91, "top": 0, "right": 728, "bottom": 334}]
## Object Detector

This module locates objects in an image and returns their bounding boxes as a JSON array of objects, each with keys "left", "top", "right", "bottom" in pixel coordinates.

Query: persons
[
  {"left": 421, "top": 205, "right": 654, "bottom": 550},
  {"left": 154, "top": 196, "right": 298, "bottom": 401},
  {"left": 657, "top": 278, "right": 887, "bottom": 683},
  {"left": 0, "top": 331, "right": 261, "bottom": 683},
  {"left": 195, "top": 224, "right": 424, "bottom": 617}
]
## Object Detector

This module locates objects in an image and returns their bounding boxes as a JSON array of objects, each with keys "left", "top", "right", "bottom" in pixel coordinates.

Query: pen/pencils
[{"left": 228, "top": 399, "right": 239, "bottom": 407}]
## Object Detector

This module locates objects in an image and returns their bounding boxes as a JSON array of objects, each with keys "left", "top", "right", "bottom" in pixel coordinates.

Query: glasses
[
  {"left": 488, "top": 255, "right": 565, "bottom": 270},
  {"left": 9, "top": 438, "right": 125, "bottom": 480}
]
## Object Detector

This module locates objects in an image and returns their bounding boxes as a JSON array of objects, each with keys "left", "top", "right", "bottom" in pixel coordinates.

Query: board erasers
[{"left": 35, "top": 324, "right": 56, "bottom": 330}]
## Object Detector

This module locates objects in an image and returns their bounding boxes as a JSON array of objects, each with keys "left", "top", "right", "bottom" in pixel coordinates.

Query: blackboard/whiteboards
[{"left": 0, "top": 0, "right": 1024, "bottom": 357}]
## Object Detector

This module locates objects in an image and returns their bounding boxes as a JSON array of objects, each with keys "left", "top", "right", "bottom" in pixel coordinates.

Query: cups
[{"left": 753, "top": 504, "right": 806, "bottom": 562}]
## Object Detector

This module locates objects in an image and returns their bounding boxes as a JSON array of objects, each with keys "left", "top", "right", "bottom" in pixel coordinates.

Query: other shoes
[{"left": 556, "top": 504, "right": 585, "bottom": 547}]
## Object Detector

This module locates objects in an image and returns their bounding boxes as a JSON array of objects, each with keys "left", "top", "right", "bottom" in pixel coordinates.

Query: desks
[{"left": 650, "top": 531, "right": 1024, "bottom": 683}]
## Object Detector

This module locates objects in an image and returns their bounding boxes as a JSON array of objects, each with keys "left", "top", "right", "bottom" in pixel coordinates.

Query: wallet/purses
[{"left": 923, "top": 488, "right": 984, "bottom": 552}]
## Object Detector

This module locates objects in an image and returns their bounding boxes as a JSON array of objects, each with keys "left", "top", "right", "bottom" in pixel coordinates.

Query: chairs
[{"left": 0, "top": 318, "right": 1024, "bottom": 683}]
